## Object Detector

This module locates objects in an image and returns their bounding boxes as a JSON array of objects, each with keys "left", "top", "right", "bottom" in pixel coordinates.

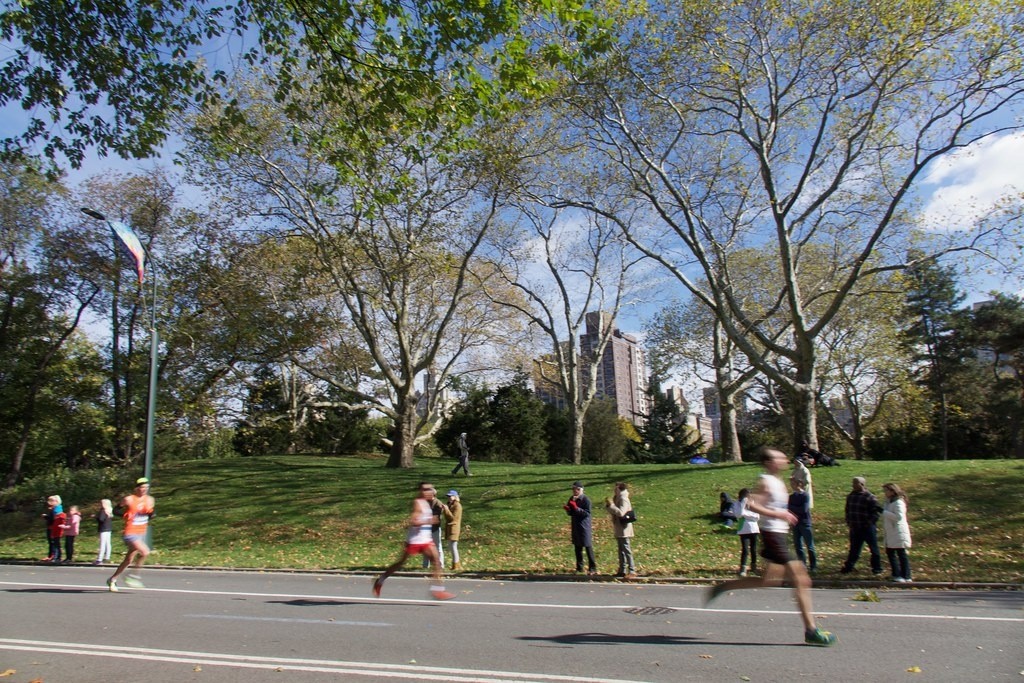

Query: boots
[
  {"left": 450, "top": 562, "right": 464, "bottom": 571},
  {"left": 740, "top": 565, "right": 746, "bottom": 576},
  {"left": 751, "top": 563, "right": 760, "bottom": 575}
]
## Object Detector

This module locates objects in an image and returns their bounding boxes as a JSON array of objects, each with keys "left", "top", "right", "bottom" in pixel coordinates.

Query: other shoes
[
  {"left": 126, "top": 578, "right": 143, "bottom": 588},
  {"left": 93, "top": 561, "right": 102, "bottom": 564},
  {"left": 435, "top": 591, "right": 457, "bottom": 599},
  {"left": 54, "top": 558, "right": 60, "bottom": 563},
  {"left": 41, "top": 555, "right": 54, "bottom": 560},
  {"left": 612, "top": 572, "right": 626, "bottom": 578},
  {"left": 372, "top": 578, "right": 382, "bottom": 596},
  {"left": 624, "top": 573, "right": 636, "bottom": 578},
  {"left": 106, "top": 580, "right": 118, "bottom": 592},
  {"left": 63, "top": 559, "right": 72, "bottom": 564},
  {"left": 103, "top": 559, "right": 110, "bottom": 563},
  {"left": 892, "top": 577, "right": 911, "bottom": 583}
]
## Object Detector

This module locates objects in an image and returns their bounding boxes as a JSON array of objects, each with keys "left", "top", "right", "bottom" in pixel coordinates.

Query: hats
[
  {"left": 574, "top": 482, "right": 582, "bottom": 488},
  {"left": 851, "top": 477, "right": 866, "bottom": 486},
  {"left": 446, "top": 490, "right": 458, "bottom": 496},
  {"left": 136, "top": 478, "right": 149, "bottom": 486}
]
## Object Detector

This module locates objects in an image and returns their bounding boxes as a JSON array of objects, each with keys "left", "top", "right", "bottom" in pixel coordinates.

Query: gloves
[
  {"left": 570, "top": 501, "right": 577, "bottom": 509},
  {"left": 564, "top": 504, "right": 571, "bottom": 511}
]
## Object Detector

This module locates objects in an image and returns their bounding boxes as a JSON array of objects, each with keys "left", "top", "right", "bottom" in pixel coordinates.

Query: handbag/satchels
[{"left": 619, "top": 511, "right": 637, "bottom": 525}]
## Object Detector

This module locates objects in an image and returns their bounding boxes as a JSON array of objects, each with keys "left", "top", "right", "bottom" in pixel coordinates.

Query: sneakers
[{"left": 806, "top": 628, "right": 834, "bottom": 644}]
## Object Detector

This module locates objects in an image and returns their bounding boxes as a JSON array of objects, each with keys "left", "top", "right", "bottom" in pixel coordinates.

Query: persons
[
  {"left": 89, "top": 498, "right": 114, "bottom": 565},
  {"left": 40, "top": 494, "right": 67, "bottom": 562},
  {"left": 372, "top": 482, "right": 458, "bottom": 601},
  {"left": 840, "top": 476, "right": 884, "bottom": 574},
  {"left": 877, "top": 482, "right": 913, "bottom": 582},
  {"left": 699, "top": 447, "right": 837, "bottom": 645},
  {"left": 451, "top": 432, "right": 474, "bottom": 477},
  {"left": 58, "top": 504, "right": 82, "bottom": 563},
  {"left": 715, "top": 441, "right": 842, "bottom": 572},
  {"left": 106, "top": 477, "right": 156, "bottom": 592},
  {"left": 605, "top": 480, "right": 638, "bottom": 578},
  {"left": 422, "top": 488, "right": 445, "bottom": 570},
  {"left": 562, "top": 481, "right": 600, "bottom": 575},
  {"left": 441, "top": 489, "right": 465, "bottom": 570}
]
